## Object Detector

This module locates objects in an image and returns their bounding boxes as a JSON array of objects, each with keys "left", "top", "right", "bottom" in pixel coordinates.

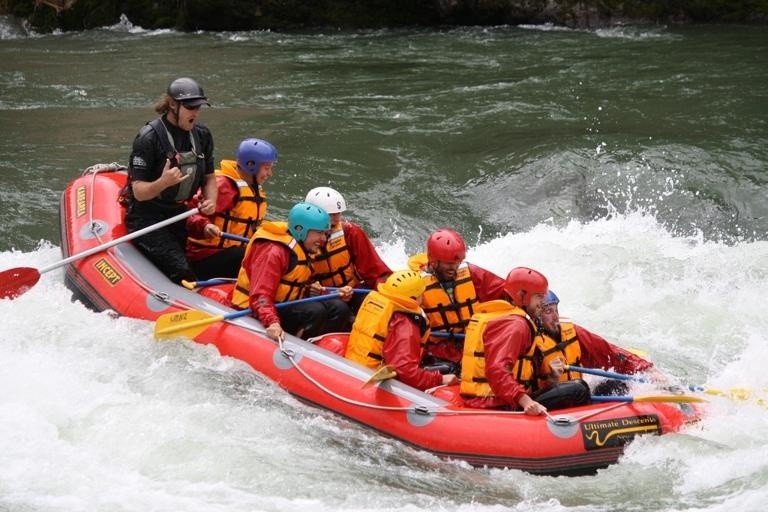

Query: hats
[{"left": 184, "top": 98, "right": 211, "bottom": 108}]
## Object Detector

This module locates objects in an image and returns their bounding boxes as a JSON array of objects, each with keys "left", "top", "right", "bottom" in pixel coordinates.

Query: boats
[{"left": 61, "top": 164, "right": 706, "bottom": 477}]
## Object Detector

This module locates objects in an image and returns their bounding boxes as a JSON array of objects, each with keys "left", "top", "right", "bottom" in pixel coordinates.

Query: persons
[
  {"left": 459, "top": 266, "right": 590, "bottom": 418},
  {"left": 231, "top": 201, "right": 351, "bottom": 340},
  {"left": 121, "top": 77, "right": 219, "bottom": 289},
  {"left": 305, "top": 185, "right": 394, "bottom": 332},
  {"left": 535, "top": 289, "right": 667, "bottom": 401},
  {"left": 185, "top": 137, "right": 279, "bottom": 280},
  {"left": 423, "top": 227, "right": 506, "bottom": 364},
  {"left": 344, "top": 269, "right": 460, "bottom": 392}
]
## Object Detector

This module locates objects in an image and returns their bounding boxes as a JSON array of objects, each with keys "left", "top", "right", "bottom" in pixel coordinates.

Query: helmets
[
  {"left": 386, "top": 271, "right": 427, "bottom": 299},
  {"left": 306, "top": 186, "right": 347, "bottom": 214},
  {"left": 427, "top": 229, "right": 466, "bottom": 269},
  {"left": 168, "top": 77, "right": 207, "bottom": 100},
  {"left": 504, "top": 267, "right": 548, "bottom": 308},
  {"left": 288, "top": 202, "right": 331, "bottom": 241},
  {"left": 237, "top": 137, "right": 278, "bottom": 176},
  {"left": 544, "top": 290, "right": 559, "bottom": 306}
]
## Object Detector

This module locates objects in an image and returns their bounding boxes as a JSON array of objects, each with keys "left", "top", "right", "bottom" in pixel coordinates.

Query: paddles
[
  {"left": 359, "top": 366, "right": 455, "bottom": 391},
  {"left": 154, "top": 287, "right": 350, "bottom": 345},
  {"left": 553, "top": 357, "right": 766, "bottom": 404},
  {"left": 0, "top": 195, "right": 206, "bottom": 301}
]
